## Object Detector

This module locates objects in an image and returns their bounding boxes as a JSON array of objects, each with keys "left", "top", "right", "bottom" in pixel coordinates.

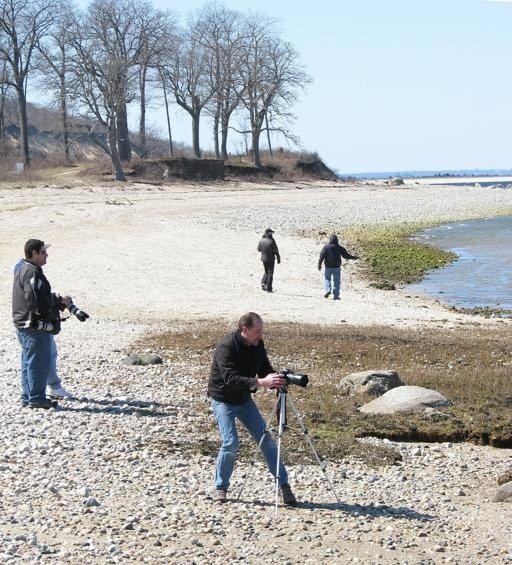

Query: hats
[
  {"left": 24, "top": 239, "right": 51, "bottom": 251},
  {"left": 265, "top": 228, "right": 275, "bottom": 234}
]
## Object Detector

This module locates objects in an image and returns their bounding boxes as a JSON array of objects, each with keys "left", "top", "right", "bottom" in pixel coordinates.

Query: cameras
[
  {"left": 277, "top": 368, "right": 310, "bottom": 387},
  {"left": 59, "top": 296, "right": 89, "bottom": 322}
]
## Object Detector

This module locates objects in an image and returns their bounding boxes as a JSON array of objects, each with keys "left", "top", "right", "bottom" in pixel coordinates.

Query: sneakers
[
  {"left": 261, "top": 283, "right": 341, "bottom": 300},
  {"left": 212, "top": 488, "right": 227, "bottom": 503},
  {"left": 281, "top": 486, "right": 297, "bottom": 504},
  {"left": 21, "top": 387, "right": 73, "bottom": 408}
]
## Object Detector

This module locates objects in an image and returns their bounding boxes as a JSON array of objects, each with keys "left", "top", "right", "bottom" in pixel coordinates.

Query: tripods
[{"left": 236, "top": 389, "right": 342, "bottom": 517}]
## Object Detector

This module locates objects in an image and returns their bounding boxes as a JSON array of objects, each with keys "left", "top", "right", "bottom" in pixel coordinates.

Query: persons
[
  {"left": 317, "top": 234, "right": 359, "bottom": 300},
  {"left": 258, "top": 229, "right": 281, "bottom": 292},
  {"left": 206, "top": 313, "right": 297, "bottom": 504},
  {"left": 11, "top": 239, "right": 57, "bottom": 409},
  {"left": 14, "top": 239, "right": 71, "bottom": 397}
]
